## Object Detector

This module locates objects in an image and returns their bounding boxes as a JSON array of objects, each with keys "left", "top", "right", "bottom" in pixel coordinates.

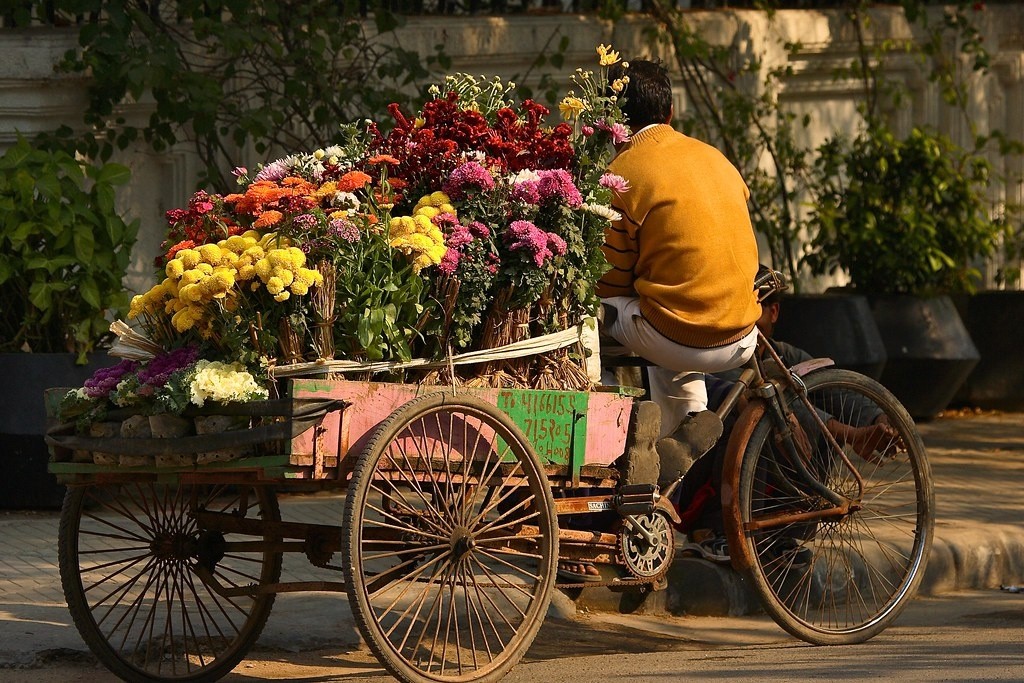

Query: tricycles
[{"left": 47, "top": 266, "right": 937, "bottom": 683}]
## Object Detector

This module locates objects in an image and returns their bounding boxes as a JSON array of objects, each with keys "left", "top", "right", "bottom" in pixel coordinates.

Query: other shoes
[
  {"left": 620, "top": 401, "right": 661, "bottom": 486},
  {"left": 655, "top": 410, "right": 723, "bottom": 490}
]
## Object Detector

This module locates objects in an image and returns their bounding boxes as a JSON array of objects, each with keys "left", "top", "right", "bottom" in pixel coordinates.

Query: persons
[
  {"left": 669, "top": 266, "right": 907, "bottom": 564},
  {"left": 591, "top": 55, "right": 761, "bottom": 486}
]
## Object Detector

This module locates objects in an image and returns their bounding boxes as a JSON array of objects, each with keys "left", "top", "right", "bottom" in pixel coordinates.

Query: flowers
[{"left": 55, "top": 43, "right": 632, "bottom": 429}]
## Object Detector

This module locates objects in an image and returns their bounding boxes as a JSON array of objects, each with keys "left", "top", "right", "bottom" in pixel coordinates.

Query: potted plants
[
  {"left": 0, "top": 126, "right": 141, "bottom": 508},
  {"left": 808, "top": 136, "right": 980, "bottom": 424}
]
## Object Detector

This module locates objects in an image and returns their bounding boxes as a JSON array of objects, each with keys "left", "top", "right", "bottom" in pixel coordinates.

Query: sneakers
[
  {"left": 681, "top": 522, "right": 731, "bottom": 560},
  {"left": 756, "top": 534, "right": 812, "bottom": 568}
]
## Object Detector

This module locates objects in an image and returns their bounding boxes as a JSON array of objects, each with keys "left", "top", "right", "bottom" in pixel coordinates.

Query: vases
[
  {"left": 118, "top": 415, "right": 155, "bottom": 467},
  {"left": 90, "top": 423, "right": 122, "bottom": 464},
  {"left": 775, "top": 294, "right": 888, "bottom": 395},
  {"left": 73, "top": 433, "right": 93, "bottom": 462},
  {"left": 194, "top": 415, "right": 254, "bottom": 464},
  {"left": 148, "top": 415, "right": 196, "bottom": 468}
]
